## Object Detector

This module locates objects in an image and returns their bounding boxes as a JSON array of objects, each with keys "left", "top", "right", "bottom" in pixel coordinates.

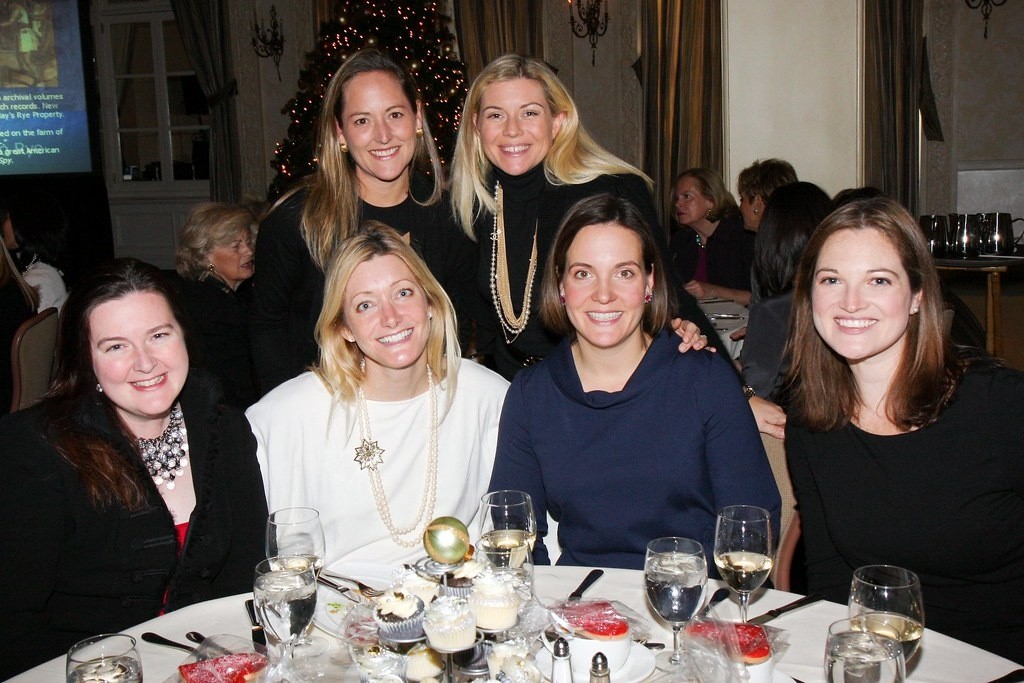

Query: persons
[
  {"left": 784, "top": 195, "right": 1024, "bottom": 667},
  {"left": 833, "top": 187, "right": 988, "bottom": 353},
  {"left": 242, "top": 219, "right": 718, "bottom": 572},
  {"left": 0, "top": 205, "right": 72, "bottom": 417},
  {"left": 681, "top": 157, "right": 798, "bottom": 304},
  {"left": 487, "top": 192, "right": 783, "bottom": 590},
  {"left": 729, "top": 182, "right": 836, "bottom": 411},
  {"left": 169, "top": 202, "right": 260, "bottom": 412},
  {"left": 0, "top": 257, "right": 276, "bottom": 683},
  {"left": 245, "top": 46, "right": 498, "bottom": 400},
  {"left": 663, "top": 167, "right": 755, "bottom": 292},
  {"left": 437, "top": 52, "right": 793, "bottom": 441}
]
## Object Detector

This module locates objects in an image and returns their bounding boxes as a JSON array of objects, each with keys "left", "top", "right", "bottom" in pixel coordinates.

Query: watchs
[{"left": 742, "top": 384, "right": 756, "bottom": 401}]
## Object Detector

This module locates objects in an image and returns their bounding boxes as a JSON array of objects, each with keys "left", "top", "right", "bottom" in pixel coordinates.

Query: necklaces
[
  {"left": 356, "top": 357, "right": 438, "bottom": 547},
  {"left": 130, "top": 404, "right": 191, "bottom": 492},
  {"left": 695, "top": 233, "right": 707, "bottom": 250},
  {"left": 490, "top": 178, "right": 539, "bottom": 345}
]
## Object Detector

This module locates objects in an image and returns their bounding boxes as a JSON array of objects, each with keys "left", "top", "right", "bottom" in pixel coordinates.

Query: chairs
[{"left": 8, "top": 306, "right": 59, "bottom": 413}]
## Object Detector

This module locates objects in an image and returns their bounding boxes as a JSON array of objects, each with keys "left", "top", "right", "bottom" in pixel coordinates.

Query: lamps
[
  {"left": 249, "top": 3, "right": 287, "bottom": 83},
  {"left": 166, "top": 76, "right": 212, "bottom": 142},
  {"left": 567, "top": 0, "right": 609, "bottom": 67}
]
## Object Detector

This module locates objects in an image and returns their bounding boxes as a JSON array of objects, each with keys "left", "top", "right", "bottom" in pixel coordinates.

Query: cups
[
  {"left": 474, "top": 537, "right": 535, "bottom": 623},
  {"left": 823, "top": 618, "right": 908, "bottom": 683},
  {"left": 479, "top": 488, "right": 537, "bottom": 571},
  {"left": 64, "top": 632, "right": 144, "bottom": 683},
  {"left": 846, "top": 565, "right": 924, "bottom": 668},
  {"left": 920, "top": 201, "right": 1024, "bottom": 261}
]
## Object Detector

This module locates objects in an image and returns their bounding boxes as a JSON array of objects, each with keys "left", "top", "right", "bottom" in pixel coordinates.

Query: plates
[
  {"left": 312, "top": 529, "right": 481, "bottom": 648},
  {"left": 531, "top": 622, "right": 658, "bottom": 683}
]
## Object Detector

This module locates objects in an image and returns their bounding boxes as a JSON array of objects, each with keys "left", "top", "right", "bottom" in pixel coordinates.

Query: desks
[
  {"left": 694, "top": 293, "right": 750, "bottom": 362},
  {"left": 1, "top": 563, "right": 1024, "bottom": 682},
  {"left": 928, "top": 254, "right": 1024, "bottom": 364}
]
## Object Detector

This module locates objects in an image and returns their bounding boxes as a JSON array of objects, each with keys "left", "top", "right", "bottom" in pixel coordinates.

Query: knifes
[
  {"left": 245, "top": 597, "right": 271, "bottom": 665},
  {"left": 745, "top": 591, "right": 827, "bottom": 625}
]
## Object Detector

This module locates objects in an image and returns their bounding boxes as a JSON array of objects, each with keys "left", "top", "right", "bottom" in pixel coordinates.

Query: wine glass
[
  {"left": 265, "top": 505, "right": 329, "bottom": 658},
  {"left": 714, "top": 504, "right": 774, "bottom": 626},
  {"left": 645, "top": 538, "right": 710, "bottom": 677},
  {"left": 254, "top": 556, "right": 317, "bottom": 667}
]
